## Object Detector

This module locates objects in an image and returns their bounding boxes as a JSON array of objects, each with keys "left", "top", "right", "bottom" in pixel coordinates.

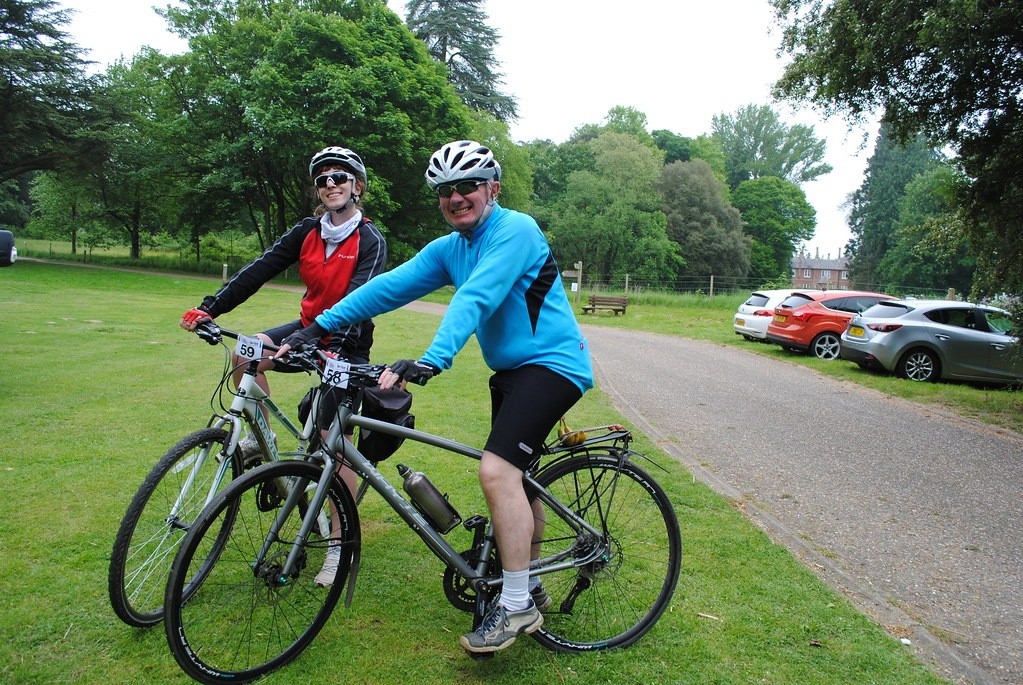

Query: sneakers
[
  {"left": 312, "top": 539, "right": 354, "bottom": 589},
  {"left": 215, "top": 426, "right": 280, "bottom": 468},
  {"left": 458, "top": 594, "right": 544, "bottom": 652},
  {"left": 486, "top": 581, "right": 553, "bottom": 613}
]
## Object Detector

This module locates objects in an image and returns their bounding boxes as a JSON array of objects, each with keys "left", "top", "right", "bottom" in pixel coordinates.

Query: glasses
[
  {"left": 436, "top": 180, "right": 487, "bottom": 198},
  {"left": 314, "top": 171, "right": 355, "bottom": 188}
]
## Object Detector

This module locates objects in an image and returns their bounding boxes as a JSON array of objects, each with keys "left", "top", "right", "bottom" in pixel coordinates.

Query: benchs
[{"left": 581, "top": 295, "right": 628, "bottom": 316}]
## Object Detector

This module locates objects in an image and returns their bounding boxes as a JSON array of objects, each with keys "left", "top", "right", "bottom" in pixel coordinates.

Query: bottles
[{"left": 396, "top": 464, "right": 462, "bottom": 535}]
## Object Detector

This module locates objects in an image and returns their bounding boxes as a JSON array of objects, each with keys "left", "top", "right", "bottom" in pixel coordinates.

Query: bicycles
[
  {"left": 162, "top": 339, "right": 683, "bottom": 685},
  {"left": 108, "top": 316, "right": 381, "bottom": 628}
]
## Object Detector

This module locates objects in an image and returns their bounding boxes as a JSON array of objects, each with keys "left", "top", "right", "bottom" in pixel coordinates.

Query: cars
[
  {"left": 839, "top": 299, "right": 1023, "bottom": 385},
  {"left": 0, "top": 230, "right": 18, "bottom": 267},
  {"left": 732, "top": 289, "right": 819, "bottom": 345},
  {"left": 767, "top": 289, "right": 901, "bottom": 360}
]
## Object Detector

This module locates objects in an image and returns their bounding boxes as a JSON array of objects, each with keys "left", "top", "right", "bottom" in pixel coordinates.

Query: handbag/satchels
[{"left": 357, "top": 384, "right": 415, "bottom": 461}]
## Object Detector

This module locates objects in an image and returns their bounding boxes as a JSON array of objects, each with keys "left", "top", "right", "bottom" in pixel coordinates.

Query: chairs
[{"left": 967, "top": 313, "right": 976, "bottom": 327}]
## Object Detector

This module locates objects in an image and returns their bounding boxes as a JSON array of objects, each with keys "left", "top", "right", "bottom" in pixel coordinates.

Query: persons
[
  {"left": 275, "top": 140, "right": 594, "bottom": 652},
  {"left": 180, "top": 147, "right": 387, "bottom": 588}
]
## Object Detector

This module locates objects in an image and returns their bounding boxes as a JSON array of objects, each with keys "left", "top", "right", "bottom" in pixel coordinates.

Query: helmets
[
  {"left": 425, "top": 140, "right": 502, "bottom": 191},
  {"left": 308, "top": 146, "right": 367, "bottom": 192}
]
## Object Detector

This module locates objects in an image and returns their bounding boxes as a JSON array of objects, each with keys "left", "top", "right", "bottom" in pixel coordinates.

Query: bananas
[{"left": 558, "top": 417, "right": 586, "bottom": 446}]
